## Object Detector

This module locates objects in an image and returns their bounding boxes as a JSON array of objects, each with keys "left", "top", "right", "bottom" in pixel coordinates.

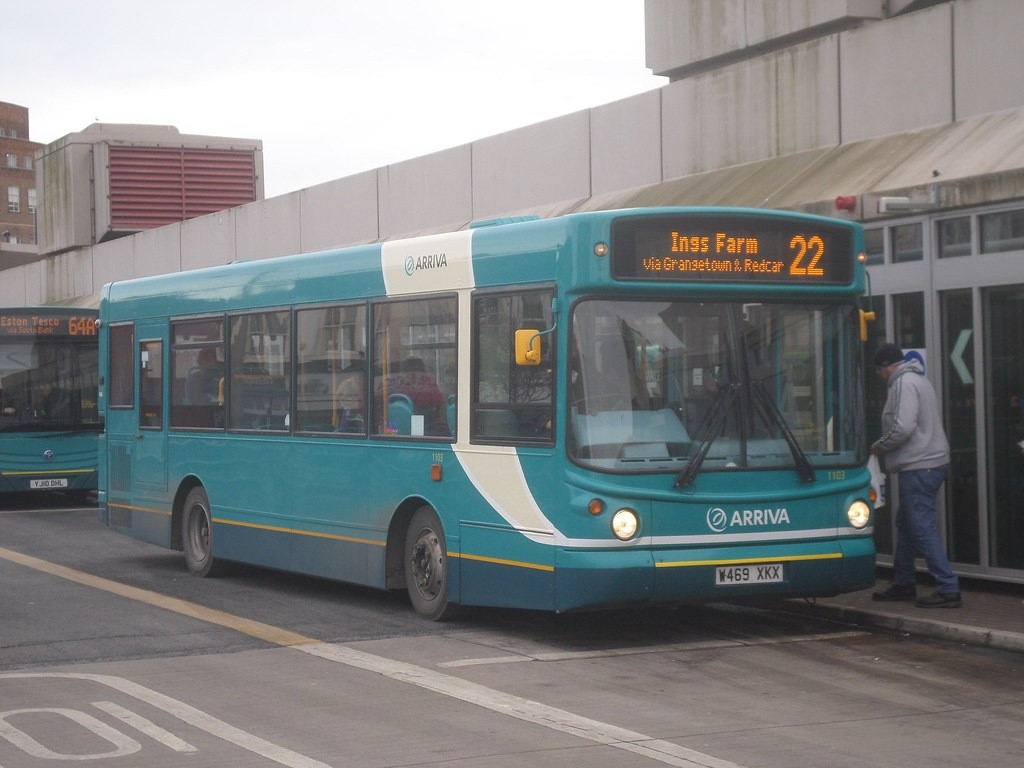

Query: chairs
[
  {"left": 446, "top": 394, "right": 458, "bottom": 437},
  {"left": 183, "top": 366, "right": 203, "bottom": 406},
  {"left": 387, "top": 393, "right": 414, "bottom": 436}
]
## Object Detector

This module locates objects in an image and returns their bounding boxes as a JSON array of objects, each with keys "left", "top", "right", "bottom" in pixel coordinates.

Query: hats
[
  {"left": 869, "top": 344, "right": 905, "bottom": 366},
  {"left": 400, "top": 354, "right": 423, "bottom": 370}
]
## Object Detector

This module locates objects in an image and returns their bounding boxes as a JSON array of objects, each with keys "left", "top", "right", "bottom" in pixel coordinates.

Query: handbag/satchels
[{"left": 866, "top": 453, "right": 887, "bottom": 509}]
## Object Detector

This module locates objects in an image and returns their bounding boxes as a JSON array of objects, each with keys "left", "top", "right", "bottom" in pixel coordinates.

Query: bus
[
  {"left": 0, "top": 306, "right": 101, "bottom": 503},
  {"left": 94, "top": 205, "right": 880, "bottom": 624}
]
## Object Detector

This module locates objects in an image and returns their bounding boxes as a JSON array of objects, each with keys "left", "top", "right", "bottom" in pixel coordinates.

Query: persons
[
  {"left": 188, "top": 348, "right": 221, "bottom": 401},
  {"left": 35, "top": 384, "right": 55, "bottom": 410},
  {"left": 870, "top": 343, "right": 961, "bottom": 607},
  {"left": 381, "top": 356, "right": 445, "bottom": 427},
  {"left": 335, "top": 376, "right": 367, "bottom": 429},
  {"left": 0, "top": 388, "right": 17, "bottom": 415}
]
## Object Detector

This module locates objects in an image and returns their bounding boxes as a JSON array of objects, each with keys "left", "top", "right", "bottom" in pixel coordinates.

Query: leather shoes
[
  {"left": 915, "top": 588, "right": 961, "bottom": 607},
  {"left": 871, "top": 585, "right": 917, "bottom": 600}
]
